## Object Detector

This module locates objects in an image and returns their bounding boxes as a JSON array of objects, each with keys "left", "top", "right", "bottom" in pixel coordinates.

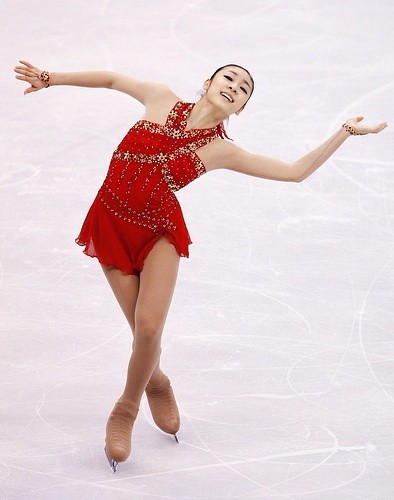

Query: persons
[{"left": 12, "top": 60, "right": 388, "bottom": 471}]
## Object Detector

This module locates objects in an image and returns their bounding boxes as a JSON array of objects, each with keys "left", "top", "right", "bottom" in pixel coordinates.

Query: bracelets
[
  {"left": 34, "top": 71, "right": 50, "bottom": 88},
  {"left": 342, "top": 123, "right": 367, "bottom": 136}
]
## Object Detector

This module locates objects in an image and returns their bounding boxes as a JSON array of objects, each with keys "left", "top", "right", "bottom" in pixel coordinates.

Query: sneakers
[
  {"left": 145, "top": 374, "right": 182, "bottom": 443},
  {"left": 106, "top": 397, "right": 140, "bottom": 471}
]
